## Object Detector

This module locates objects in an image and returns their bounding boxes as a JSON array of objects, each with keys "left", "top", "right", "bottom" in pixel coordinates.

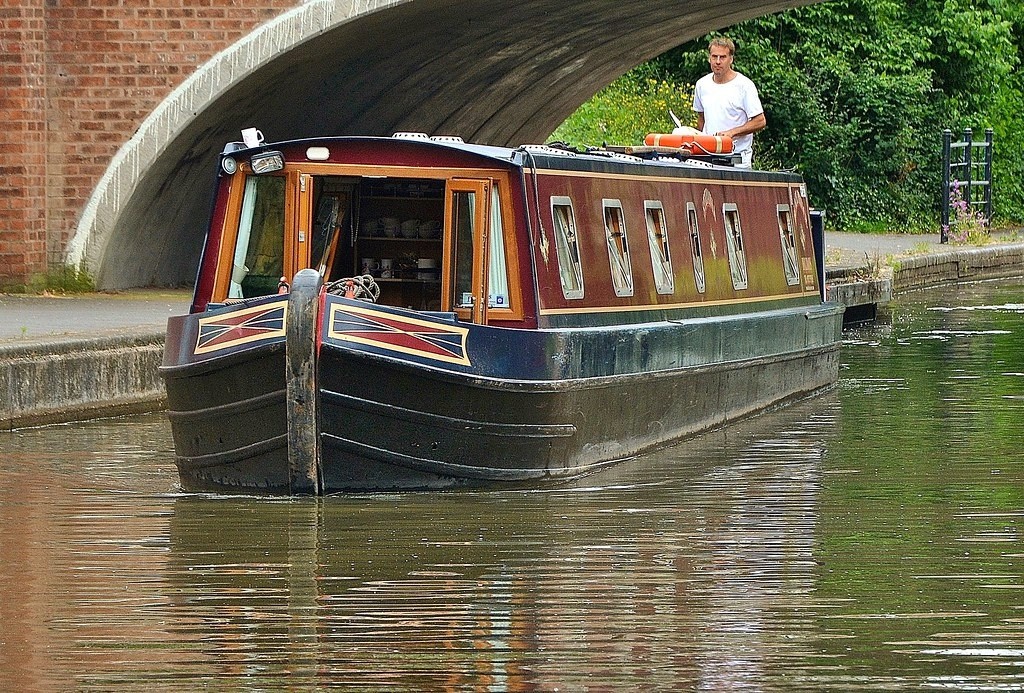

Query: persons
[{"left": 693, "top": 39, "right": 766, "bottom": 170}]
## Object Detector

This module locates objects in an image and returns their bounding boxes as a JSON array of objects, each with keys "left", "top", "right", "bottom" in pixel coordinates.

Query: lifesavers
[{"left": 646, "top": 133, "right": 732, "bottom": 155}]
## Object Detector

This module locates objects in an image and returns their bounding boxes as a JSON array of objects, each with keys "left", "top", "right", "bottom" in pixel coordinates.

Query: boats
[{"left": 155, "top": 126, "right": 847, "bottom": 498}]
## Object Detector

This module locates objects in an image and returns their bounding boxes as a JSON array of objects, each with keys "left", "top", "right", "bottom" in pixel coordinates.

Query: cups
[
  {"left": 417, "top": 259, "right": 436, "bottom": 279},
  {"left": 363, "top": 217, "right": 440, "bottom": 238},
  {"left": 381, "top": 259, "right": 393, "bottom": 278},
  {"left": 361, "top": 257, "right": 380, "bottom": 276}
]
